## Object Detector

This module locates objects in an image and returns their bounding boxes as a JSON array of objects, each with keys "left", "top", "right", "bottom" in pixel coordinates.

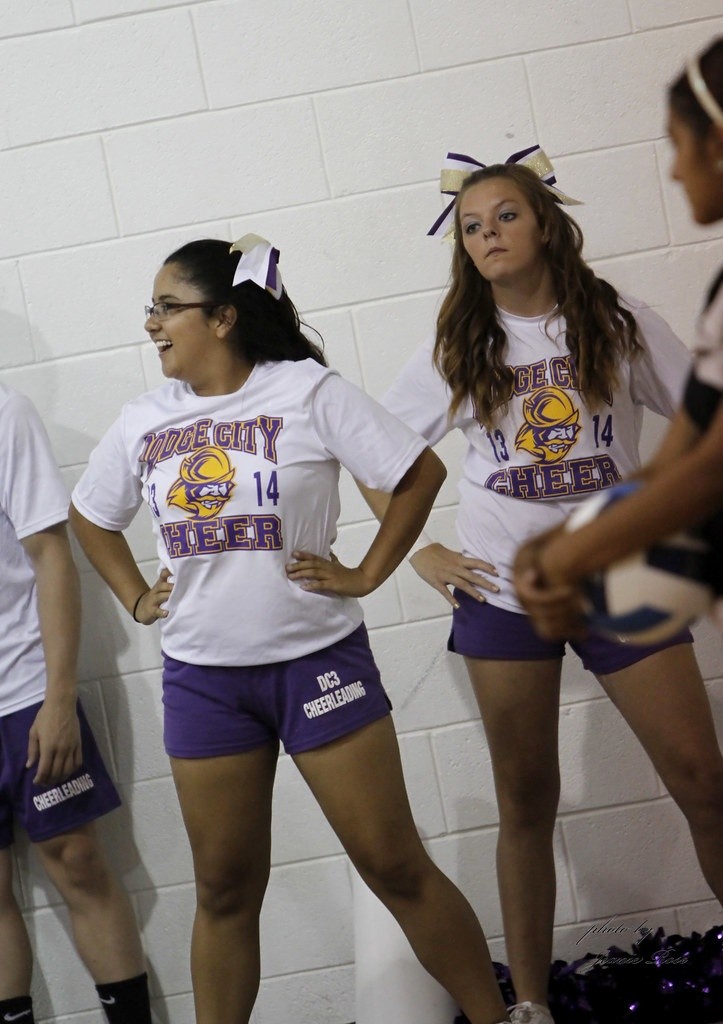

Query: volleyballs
[{"left": 560, "top": 478, "right": 716, "bottom": 645}]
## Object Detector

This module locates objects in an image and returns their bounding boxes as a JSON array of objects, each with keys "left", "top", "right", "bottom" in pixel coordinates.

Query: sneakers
[{"left": 505, "top": 1001, "right": 555, "bottom": 1024}]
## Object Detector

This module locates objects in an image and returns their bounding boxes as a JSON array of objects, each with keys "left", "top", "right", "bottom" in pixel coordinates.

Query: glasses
[{"left": 144, "top": 301, "right": 233, "bottom": 321}]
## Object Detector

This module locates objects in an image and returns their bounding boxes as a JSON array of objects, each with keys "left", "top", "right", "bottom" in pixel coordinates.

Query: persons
[
  {"left": 68, "top": 236, "right": 509, "bottom": 1024},
  {"left": 353, "top": 145, "right": 723, "bottom": 1024},
  {"left": 511, "top": 37, "right": 723, "bottom": 646},
  {"left": 0, "top": 382, "right": 153, "bottom": 1024}
]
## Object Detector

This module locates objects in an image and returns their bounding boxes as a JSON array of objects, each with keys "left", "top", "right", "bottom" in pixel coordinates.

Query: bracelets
[{"left": 133, "top": 591, "right": 147, "bottom": 622}]
